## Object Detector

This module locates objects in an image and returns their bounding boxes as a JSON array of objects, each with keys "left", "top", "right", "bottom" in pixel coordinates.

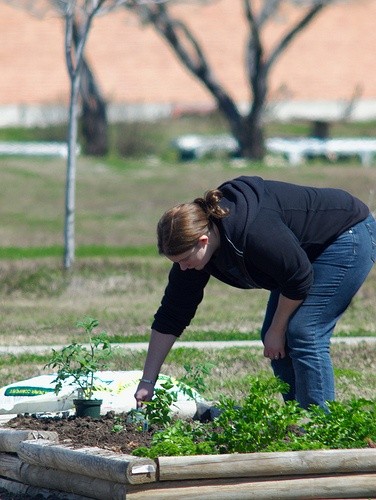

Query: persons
[{"left": 133, "top": 175, "right": 376, "bottom": 414}]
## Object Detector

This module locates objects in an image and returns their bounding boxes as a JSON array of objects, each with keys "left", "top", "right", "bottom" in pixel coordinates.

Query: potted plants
[{"left": 43, "top": 315, "right": 114, "bottom": 419}]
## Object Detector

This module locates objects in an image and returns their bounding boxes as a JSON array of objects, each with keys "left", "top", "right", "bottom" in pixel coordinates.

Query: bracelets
[{"left": 139, "top": 379, "right": 156, "bottom": 384}]
